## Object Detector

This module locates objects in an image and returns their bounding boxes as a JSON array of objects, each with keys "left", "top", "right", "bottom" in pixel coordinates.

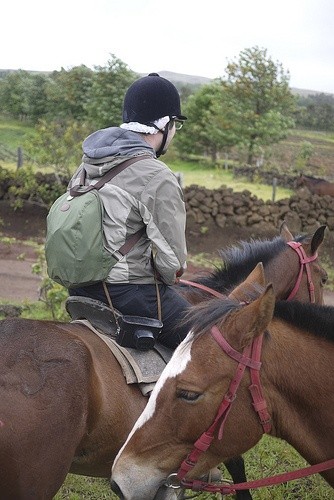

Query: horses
[
  {"left": 0, "top": 222, "right": 329, "bottom": 500},
  {"left": 110, "top": 260, "right": 334, "bottom": 500}
]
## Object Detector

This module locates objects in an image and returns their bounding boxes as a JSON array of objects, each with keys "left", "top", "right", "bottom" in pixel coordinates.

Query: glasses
[{"left": 172, "top": 119, "right": 184, "bottom": 130}]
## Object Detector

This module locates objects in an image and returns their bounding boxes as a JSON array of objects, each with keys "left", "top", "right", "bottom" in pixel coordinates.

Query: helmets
[{"left": 122, "top": 73, "right": 187, "bottom": 123}]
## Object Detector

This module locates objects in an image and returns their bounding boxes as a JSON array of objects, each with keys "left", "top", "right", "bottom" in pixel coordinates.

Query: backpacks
[{"left": 44, "top": 154, "right": 154, "bottom": 288}]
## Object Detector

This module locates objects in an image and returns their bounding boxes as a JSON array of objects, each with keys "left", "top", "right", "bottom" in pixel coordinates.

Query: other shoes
[{"left": 197, "top": 467, "right": 223, "bottom": 483}]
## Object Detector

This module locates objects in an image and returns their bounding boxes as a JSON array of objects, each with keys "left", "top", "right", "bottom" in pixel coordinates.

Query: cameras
[{"left": 116, "top": 315, "right": 164, "bottom": 351}]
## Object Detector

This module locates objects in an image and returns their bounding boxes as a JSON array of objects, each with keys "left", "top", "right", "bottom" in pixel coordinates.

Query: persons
[{"left": 64, "top": 72, "right": 204, "bottom": 349}]
